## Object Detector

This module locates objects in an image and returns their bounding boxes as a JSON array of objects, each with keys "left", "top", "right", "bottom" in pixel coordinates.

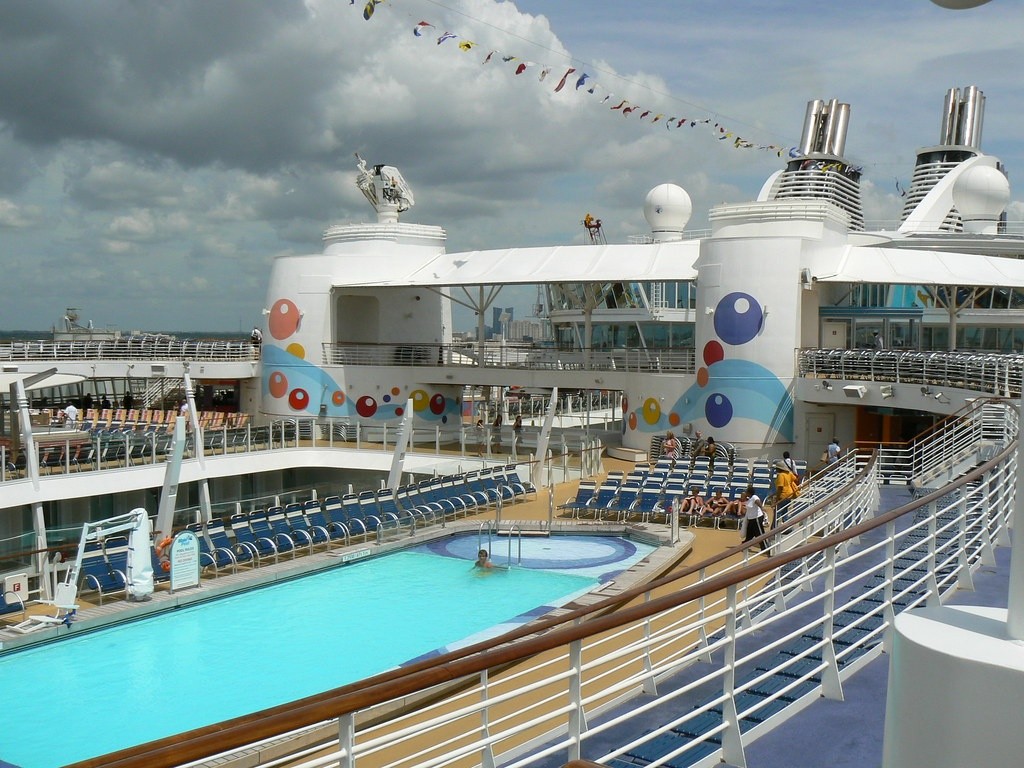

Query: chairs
[
  {"left": 77, "top": 462, "right": 538, "bottom": 606},
  {"left": 0, "top": 586, "right": 27, "bottom": 624},
  {"left": 4, "top": 415, "right": 360, "bottom": 480},
  {"left": 797, "top": 345, "right": 1023, "bottom": 396},
  {"left": 555, "top": 434, "right": 807, "bottom": 532}
]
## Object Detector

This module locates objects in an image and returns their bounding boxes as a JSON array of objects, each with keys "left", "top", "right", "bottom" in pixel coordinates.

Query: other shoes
[{"left": 782, "top": 530, "right": 792, "bottom": 535}]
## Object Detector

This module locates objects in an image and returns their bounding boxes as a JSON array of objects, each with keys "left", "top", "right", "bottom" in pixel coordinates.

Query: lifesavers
[{"left": 156, "top": 538, "right": 174, "bottom": 559}]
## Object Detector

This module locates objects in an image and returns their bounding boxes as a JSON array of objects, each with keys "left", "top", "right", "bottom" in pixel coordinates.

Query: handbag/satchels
[
  {"left": 740, "top": 514, "right": 749, "bottom": 538},
  {"left": 792, "top": 482, "right": 802, "bottom": 499},
  {"left": 761, "top": 512, "right": 770, "bottom": 528},
  {"left": 820, "top": 452, "right": 828, "bottom": 464}
]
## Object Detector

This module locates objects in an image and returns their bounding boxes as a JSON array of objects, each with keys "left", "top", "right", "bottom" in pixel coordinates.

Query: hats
[
  {"left": 833, "top": 436, "right": 840, "bottom": 442},
  {"left": 770, "top": 462, "right": 789, "bottom": 471}
]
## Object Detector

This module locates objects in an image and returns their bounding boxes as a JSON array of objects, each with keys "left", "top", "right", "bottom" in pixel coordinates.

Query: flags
[
  {"left": 363, "top": 0, "right": 384, "bottom": 21},
  {"left": 413, "top": 21, "right": 863, "bottom": 175}
]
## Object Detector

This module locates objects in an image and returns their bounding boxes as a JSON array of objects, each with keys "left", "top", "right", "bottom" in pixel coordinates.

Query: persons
[
  {"left": 742, "top": 486, "right": 768, "bottom": 554},
  {"left": 691, "top": 430, "right": 715, "bottom": 467},
  {"left": 251, "top": 326, "right": 262, "bottom": 347},
  {"left": 513, "top": 415, "right": 523, "bottom": 455},
  {"left": 476, "top": 420, "right": 485, "bottom": 457},
  {"left": 101, "top": 396, "right": 111, "bottom": 409},
  {"left": 827, "top": 437, "right": 840, "bottom": 465},
  {"left": 680, "top": 486, "right": 747, "bottom": 516},
  {"left": 220, "top": 391, "right": 226, "bottom": 401},
  {"left": 123, "top": 391, "right": 132, "bottom": 410},
  {"left": 770, "top": 461, "right": 798, "bottom": 530},
  {"left": 64, "top": 400, "right": 77, "bottom": 429},
  {"left": 663, "top": 430, "right": 676, "bottom": 457},
  {"left": 173, "top": 398, "right": 188, "bottom": 417},
  {"left": 491, "top": 414, "right": 503, "bottom": 453},
  {"left": 475, "top": 549, "right": 491, "bottom": 568},
  {"left": 782, "top": 451, "right": 798, "bottom": 476},
  {"left": 85, "top": 393, "right": 93, "bottom": 411},
  {"left": 872, "top": 329, "right": 883, "bottom": 349}
]
